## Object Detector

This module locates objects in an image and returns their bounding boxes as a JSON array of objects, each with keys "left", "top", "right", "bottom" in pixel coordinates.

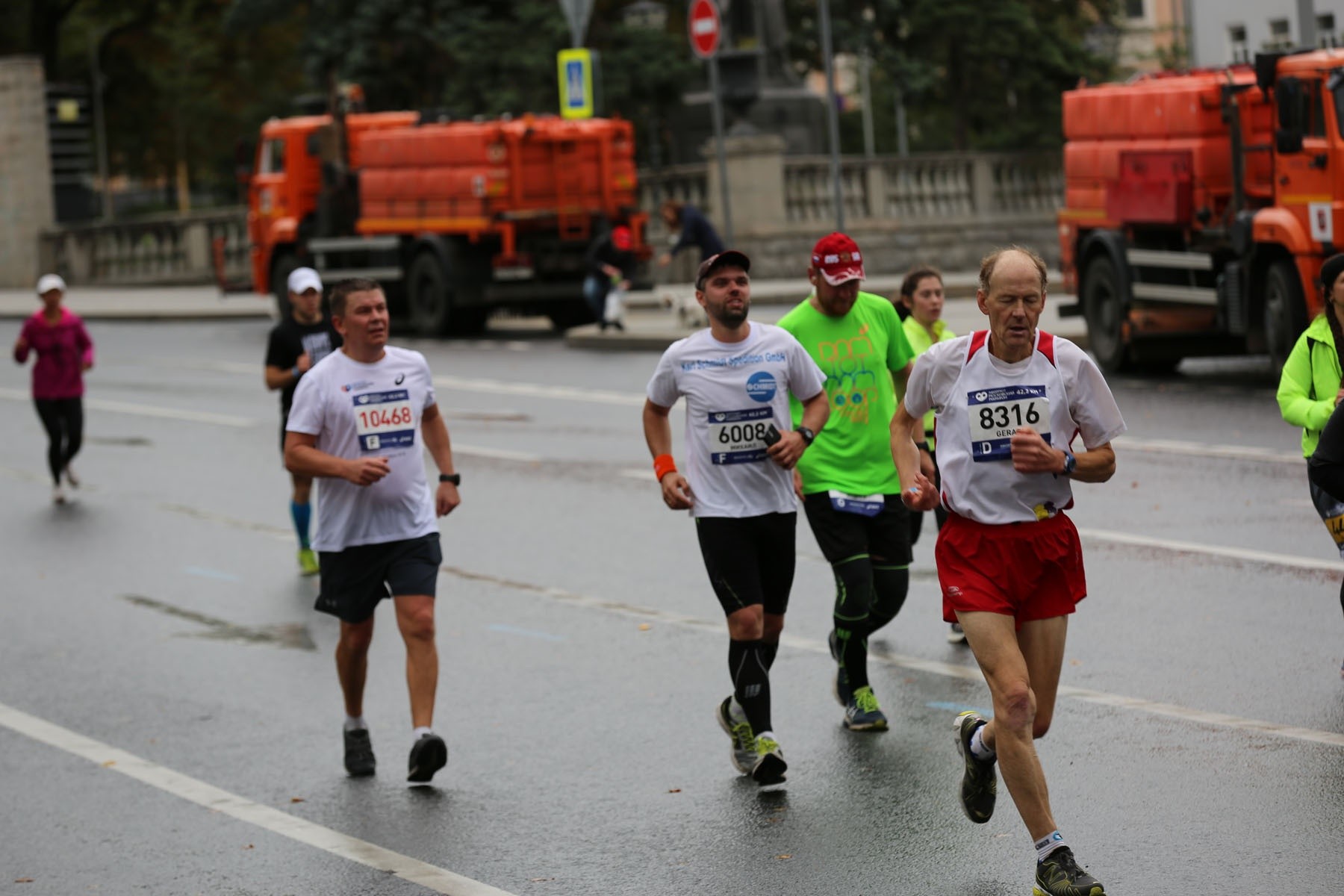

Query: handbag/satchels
[{"left": 604, "top": 292, "right": 630, "bottom": 323}]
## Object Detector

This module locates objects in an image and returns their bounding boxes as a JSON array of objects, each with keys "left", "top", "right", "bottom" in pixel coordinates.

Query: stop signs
[{"left": 686, "top": 0, "right": 721, "bottom": 60}]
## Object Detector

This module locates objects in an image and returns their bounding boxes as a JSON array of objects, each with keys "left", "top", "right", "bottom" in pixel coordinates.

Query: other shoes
[
  {"left": 947, "top": 622, "right": 968, "bottom": 643},
  {"left": 343, "top": 722, "right": 376, "bottom": 778},
  {"left": 53, "top": 485, "right": 65, "bottom": 504},
  {"left": 407, "top": 733, "right": 447, "bottom": 782},
  {"left": 297, "top": 548, "right": 320, "bottom": 576},
  {"left": 65, "top": 467, "right": 80, "bottom": 488}
]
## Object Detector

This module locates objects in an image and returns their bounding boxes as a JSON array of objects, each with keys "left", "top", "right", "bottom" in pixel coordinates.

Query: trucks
[
  {"left": 247, "top": 80, "right": 656, "bottom": 339},
  {"left": 1058, "top": 35, "right": 1344, "bottom": 377}
]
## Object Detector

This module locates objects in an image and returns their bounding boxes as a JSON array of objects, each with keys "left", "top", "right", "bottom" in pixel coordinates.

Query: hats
[
  {"left": 36, "top": 273, "right": 67, "bottom": 296},
  {"left": 613, "top": 226, "right": 633, "bottom": 250},
  {"left": 287, "top": 267, "right": 323, "bottom": 295},
  {"left": 811, "top": 231, "right": 866, "bottom": 286},
  {"left": 695, "top": 251, "right": 751, "bottom": 289}
]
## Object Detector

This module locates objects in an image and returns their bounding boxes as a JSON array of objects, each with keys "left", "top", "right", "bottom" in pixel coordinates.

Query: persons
[
  {"left": 892, "top": 270, "right": 969, "bottom": 644},
  {"left": 775, "top": 232, "right": 936, "bottom": 732},
  {"left": 641, "top": 250, "right": 831, "bottom": 784},
  {"left": 586, "top": 223, "right": 635, "bottom": 332},
  {"left": 285, "top": 278, "right": 462, "bottom": 785},
  {"left": 14, "top": 273, "right": 95, "bottom": 506},
  {"left": 265, "top": 267, "right": 346, "bottom": 577},
  {"left": 890, "top": 250, "right": 1128, "bottom": 896},
  {"left": 1277, "top": 254, "right": 1344, "bottom": 610},
  {"left": 653, "top": 200, "right": 728, "bottom": 267}
]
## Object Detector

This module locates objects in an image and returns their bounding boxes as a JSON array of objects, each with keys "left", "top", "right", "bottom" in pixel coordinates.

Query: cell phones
[{"left": 763, "top": 423, "right": 782, "bottom": 455}]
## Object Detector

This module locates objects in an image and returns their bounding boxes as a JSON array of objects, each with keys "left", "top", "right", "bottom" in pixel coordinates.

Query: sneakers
[
  {"left": 953, "top": 710, "right": 997, "bottom": 824},
  {"left": 843, "top": 686, "right": 887, "bottom": 732},
  {"left": 828, "top": 630, "right": 851, "bottom": 707},
  {"left": 716, "top": 695, "right": 759, "bottom": 774},
  {"left": 1033, "top": 846, "right": 1107, "bottom": 896},
  {"left": 750, "top": 730, "right": 788, "bottom": 787}
]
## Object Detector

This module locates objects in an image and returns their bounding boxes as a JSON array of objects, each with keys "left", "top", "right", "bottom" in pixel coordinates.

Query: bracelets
[
  {"left": 437, "top": 472, "right": 461, "bottom": 486},
  {"left": 916, "top": 440, "right": 931, "bottom": 452},
  {"left": 899, "top": 487, "right": 917, "bottom": 503},
  {"left": 653, "top": 454, "right": 678, "bottom": 483},
  {"left": 291, "top": 365, "right": 301, "bottom": 380}
]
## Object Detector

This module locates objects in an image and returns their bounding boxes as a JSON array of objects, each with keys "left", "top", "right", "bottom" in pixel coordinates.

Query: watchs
[
  {"left": 797, "top": 426, "right": 813, "bottom": 446},
  {"left": 1061, "top": 447, "right": 1076, "bottom": 478}
]
